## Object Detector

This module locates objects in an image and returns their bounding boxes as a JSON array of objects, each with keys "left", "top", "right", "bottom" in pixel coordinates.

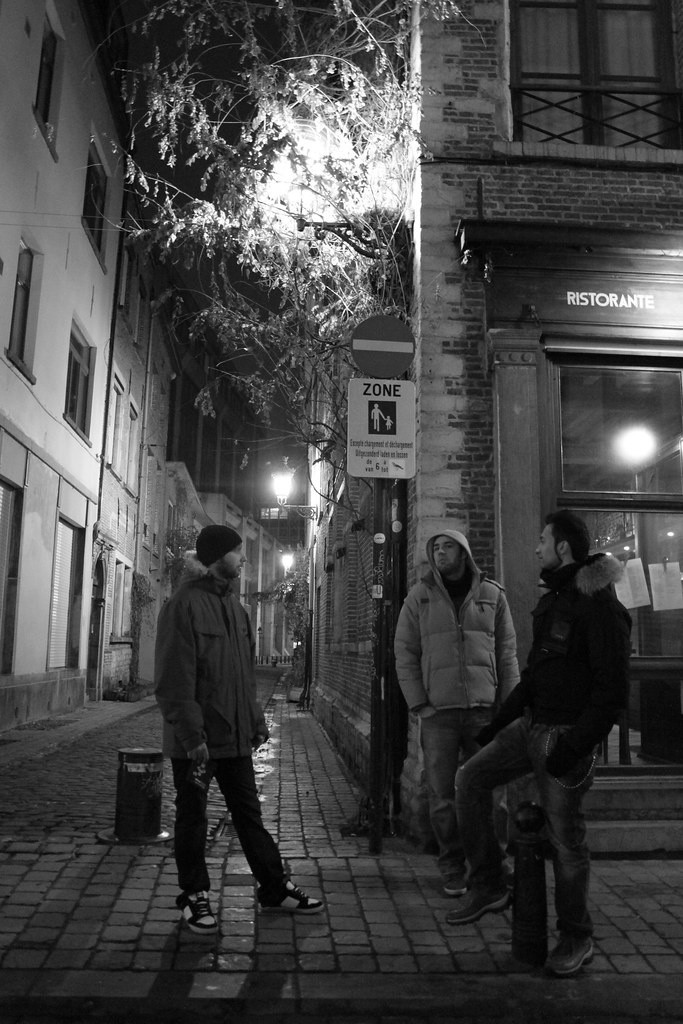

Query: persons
[
  {"left": 393, "top": 530, "right": 520, "bottom": 896},
  {"left": 446, "top": 509, "right": 633, "bottom": 978},
  {"left": 153, "top": 525, "right": 324, "bottom": 933}
]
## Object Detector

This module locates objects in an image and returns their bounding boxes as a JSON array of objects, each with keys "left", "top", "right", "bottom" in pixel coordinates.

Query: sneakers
[
  {"left": 445, "top": 879, "right": 510, "bottom": 927},
  {"left": 256, "top": 881, "right": 325, "bottom": 915},
  {"left": 544, "top": 934, "right": 594, "bottom": 978},
  {"left": 176, "top": 892, "right": 219, "bottom": 936},
  {"left": 442, "top": 877, "right": 467, "bottom": 896}
]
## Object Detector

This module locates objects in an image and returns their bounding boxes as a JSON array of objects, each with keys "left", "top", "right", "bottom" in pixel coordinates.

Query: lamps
[
  {"left": 271, "top": 455, "right": 318, "bottom": 522},
  {"left": 336, "top": 547, "right": 346, "bottom": 558},
  {"left": 325, "top": 564, "right": 335, "bottom": 573},
  {"left": 352, "top": 518, "right": 365, "bottom": 531},
  {"left": 257, "top": 625, "right": 263, "bottom": 634}
]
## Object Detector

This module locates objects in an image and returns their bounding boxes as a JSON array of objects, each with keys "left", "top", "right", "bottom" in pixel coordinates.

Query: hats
[{"left": 195, "top": 524, "right": 242, "bottom": 568}]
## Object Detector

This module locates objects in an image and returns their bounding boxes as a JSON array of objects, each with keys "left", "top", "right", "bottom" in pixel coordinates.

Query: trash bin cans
[{"left": 95, "top": 746, "right": 172, "bottom": 843}]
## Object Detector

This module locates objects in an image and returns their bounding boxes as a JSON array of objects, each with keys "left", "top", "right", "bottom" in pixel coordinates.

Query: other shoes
[{"left": 506, "top": 879, "right": 514, "bottom": 889}]
